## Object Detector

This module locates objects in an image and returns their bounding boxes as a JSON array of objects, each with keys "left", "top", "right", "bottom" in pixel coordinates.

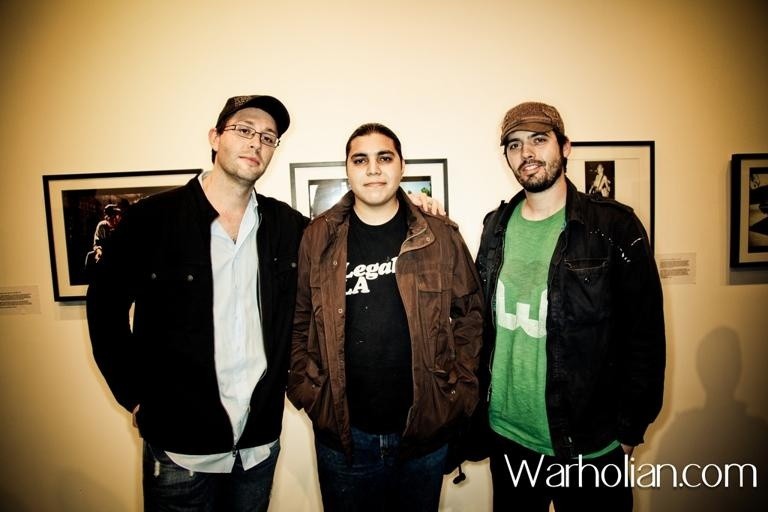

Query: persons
[
  {"left": 87, "top": 96, "right": 446, "bottom": 510},
  {"left": 92, "top": 202, "right": 121, "bottom": 261},
  {"left": 475, "top": 103, "right": 666, "bottom": 511},
  {"left": 288, "top": 123, "right": 486, "bottom": 512},
  {"left": 590, "top": 163, "right": 612, "bottom": 198}
]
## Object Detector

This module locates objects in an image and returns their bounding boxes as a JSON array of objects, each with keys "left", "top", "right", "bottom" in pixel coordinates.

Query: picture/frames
[
  {"left": 289, "top": 158, "right": 448, "bottom": 221},
  {"left": 568, "top": 141, "right": 656, "bottom": 251},
  {"left": 41, "top": 170, "right": 206, "bottom": 304},
  {"left": 729, "top": 152, "right": 767, "bottom": 271}
]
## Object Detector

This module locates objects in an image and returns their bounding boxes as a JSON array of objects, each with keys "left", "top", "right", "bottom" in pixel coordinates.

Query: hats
[
  {"left": 500, "top": 101, "right": 564, "bottom": 146},
  {"left": 216, "top": 94, "right": 290, "bottom": 136},
  {"left": 104, "top": 204, "right": 121, "bottom": 214}
]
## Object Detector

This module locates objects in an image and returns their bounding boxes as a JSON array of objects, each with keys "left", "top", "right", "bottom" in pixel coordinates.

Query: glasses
[{"left": 223, "top": 123, "right": 283, "bottom": 149}]
